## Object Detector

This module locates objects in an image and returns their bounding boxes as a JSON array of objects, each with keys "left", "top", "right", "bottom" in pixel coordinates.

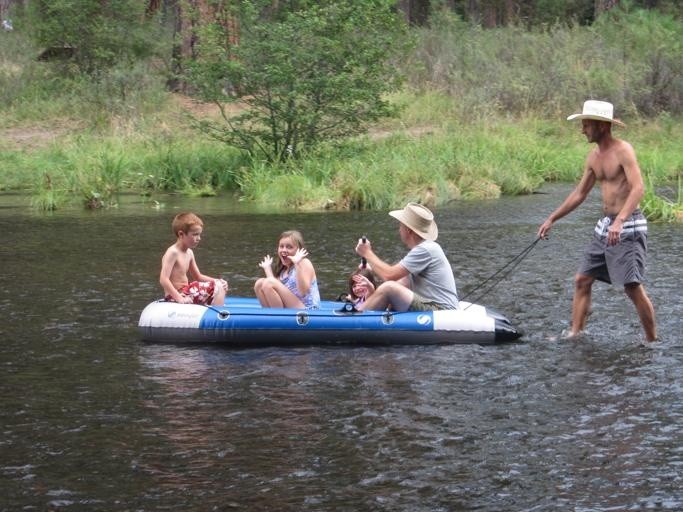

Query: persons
[
  {"left": 348, "top": 270, "right": 376, "bottom": 304},
  {"left": 538, "top": 98, "right": 656, "bottom": 342},
  {"left": 254, "top": 230, "right": 320, "bottom": 308},
  {"left": 356, "top": 201, "right": 457, "bottom": 311},
  {"left": 160, "top": 211, "right": 228, "bottom": 306}
]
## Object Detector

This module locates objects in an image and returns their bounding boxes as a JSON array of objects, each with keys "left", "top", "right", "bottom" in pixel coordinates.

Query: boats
[{"left": 137, "top": 295, "right": 523, "bottom": 347}]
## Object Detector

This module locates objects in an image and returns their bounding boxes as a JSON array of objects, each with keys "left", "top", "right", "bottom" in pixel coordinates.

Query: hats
[
  {"left": 567, "top": 100, "right": 626, "bottom": 128},
  {"left": 389, "top": 203, "right": 438, "bottom": 240}
]
